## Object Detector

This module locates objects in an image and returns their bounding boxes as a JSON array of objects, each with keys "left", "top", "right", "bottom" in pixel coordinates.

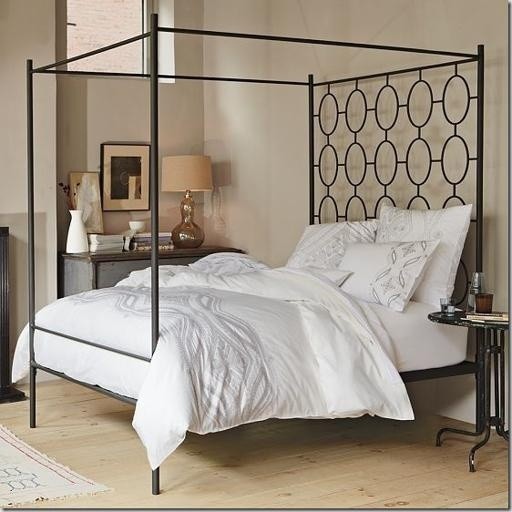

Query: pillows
[
  {"left": 283, "top": 217, "right": 380, "bottom": 286},
  {"left": 333, "top": 237, "right": 441, "bottom": 315},
  {"left": 372, "top": 200, "right": 475, "bottom": 311},
  {"left": 188, "top": 252, "right": 354, "bottom": 287}
]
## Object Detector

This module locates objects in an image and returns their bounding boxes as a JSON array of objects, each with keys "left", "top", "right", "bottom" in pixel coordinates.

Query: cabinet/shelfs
[{"left": 58, "top": 244, "right": 249, "bottom": 298}]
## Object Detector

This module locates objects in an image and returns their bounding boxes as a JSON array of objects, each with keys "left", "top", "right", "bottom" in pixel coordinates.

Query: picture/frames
[
  {"left": 99, "top": 140, "right": 152, "bottom": 214},
  {"left": 68, "top": 171, "right": 105, "bottom": 235}
]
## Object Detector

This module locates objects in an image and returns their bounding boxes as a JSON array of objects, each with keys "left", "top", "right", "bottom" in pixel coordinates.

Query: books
[
  {"left": 466, "top": 311, "right": 509, "bottom": 322},
  {"left": 88, "top": 232, "right": 125, "bottom": 255},
  {"left": 132, "top": 231, "right": 175, "bottom": 251}
]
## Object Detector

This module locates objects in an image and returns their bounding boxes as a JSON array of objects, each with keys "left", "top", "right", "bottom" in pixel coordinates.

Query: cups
[
  {"left": 440, "top": 297, "right": 456, "bottom": 318},
  {"left": 475, "top": 293, "right": 492, "bottom": 312},
  {"left": 129, "top": 221, "right": 146, "bottom": 233}
]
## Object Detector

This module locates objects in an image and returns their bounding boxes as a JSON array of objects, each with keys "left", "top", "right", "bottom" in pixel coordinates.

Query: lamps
[{"left": 159, "top": 153, "right": 220, "bottom": 248}]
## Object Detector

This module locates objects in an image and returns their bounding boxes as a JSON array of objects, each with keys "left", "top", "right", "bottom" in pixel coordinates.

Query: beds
[{"left": 14, "top": 12, "right": 488, "bottom": 498}]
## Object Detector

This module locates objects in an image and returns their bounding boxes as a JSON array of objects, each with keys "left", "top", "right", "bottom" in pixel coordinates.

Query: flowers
[{"left": 57, "top": 181, "right": 80, "bottom": 210}]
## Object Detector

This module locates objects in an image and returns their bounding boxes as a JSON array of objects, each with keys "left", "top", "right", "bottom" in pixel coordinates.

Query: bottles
[{"left": 466, "top": 272, "right": 487, "bottom": 312}]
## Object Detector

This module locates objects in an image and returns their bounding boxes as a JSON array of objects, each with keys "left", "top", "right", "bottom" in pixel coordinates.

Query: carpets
[{"left": 0, "top": 424, "right": 112, "bottom": 507}]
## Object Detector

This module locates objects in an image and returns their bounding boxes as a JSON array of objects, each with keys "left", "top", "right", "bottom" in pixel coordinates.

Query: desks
[{"left": 429, "top": 307, "right": 510, "bottom": 474}]
